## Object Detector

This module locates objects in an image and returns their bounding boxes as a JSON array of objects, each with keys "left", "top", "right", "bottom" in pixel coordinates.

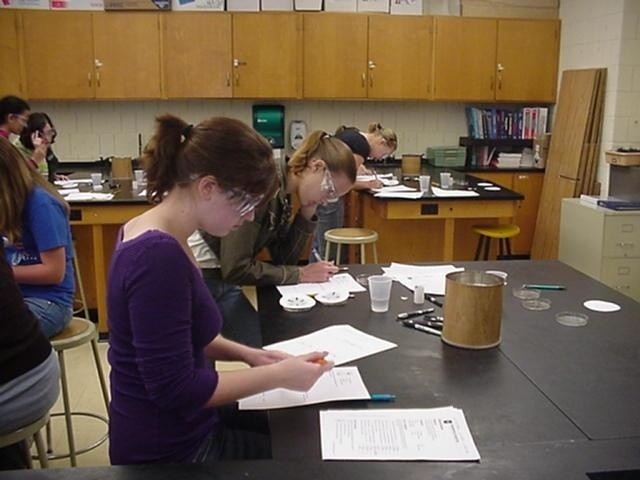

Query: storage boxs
[
  {"left": 102, "top": 0, "right": 171, "bottom": 13},
  {"left": 259, "top": 0, "right": 296, "bottom": 14},
  {"left": 289, "top": 1, "right": 325, "bottom": 11},
  {"left": 386, "top": 1, "right": 422, "bottom": 16},
  {"left": 166, "top": 0, "right": 228, "bottom": 14},
  {"left": 416, "top": 0, "right": 459, "bottom": 16},
  {"left": 319, "top": 1, "right": 358, "bottom": 13},
  {"left": 353, "top": 1, "right": 392, "bottom": 15},
  {"left": 222, "top": 0, "right": 263, "bottom": 13}
]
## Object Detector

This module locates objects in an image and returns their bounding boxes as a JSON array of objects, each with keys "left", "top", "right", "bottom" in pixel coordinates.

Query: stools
[
  {"left": 473, "top": 221, "right": 520, "bottom": 260},
  {"left": 32, "top": 314, "right": 113, "bottom": 468},
  {"left": 66, "top": 236, "right": 93, "bottom": 322},
  {"left": 324, "top": 227, "right": 378, "bottom": 265},
  {"left": 0, "top": 404, "right": 57, "bottom": 470}
]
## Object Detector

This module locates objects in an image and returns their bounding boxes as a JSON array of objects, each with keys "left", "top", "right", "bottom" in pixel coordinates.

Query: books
[
  {"left": 464, "top": 106, "right": 548, "bottom": 139},
  {"left": 470, "top": 148, "right": 522, "bottom": 168},
  {"left": 597, "top": 198, "right": 639, "bottom": 212}
]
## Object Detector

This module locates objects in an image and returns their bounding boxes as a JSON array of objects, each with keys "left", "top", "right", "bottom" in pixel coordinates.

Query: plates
[
  {"left": 511, "top": 287, "right": 543, "bottom": 300},
  {"left": 555, "top": 311, "right": 589, "bottom": 327},
  {"left": 522, "top": 297, "right": 552, "bottom": 312}
]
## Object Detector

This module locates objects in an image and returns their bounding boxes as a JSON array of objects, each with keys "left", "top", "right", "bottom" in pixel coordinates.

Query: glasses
[
  {"left": 193, "top": 172, "right": 263, "bottom": 216},
  {"left": 312, "top": 159, "right": 338, "bottom": 203},
  {"left": 38, "top": 129, "right": 55, "bottom": 137},
  {"left": 14, "top": 113, "right": 28, "bottom": 126},
  {"left": 381, "top": 153, "right": 388, "bottom": 160}
]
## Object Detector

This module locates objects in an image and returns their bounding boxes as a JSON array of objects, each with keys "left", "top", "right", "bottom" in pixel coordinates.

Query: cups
[
  {"left": 419, "top": 175, "right": 431, "bottom": 192},
  {"left": 135, "top": 170, "right": 144, "bottom": 184},
  {"left": 440, "top": 172, "right": 451, "bottom": 188},
  {"left": 91, "top": 173, "right": 102, "bottom": 187},
  {"left": 367, "top": 275, "right": 393, "bottom": 313}
]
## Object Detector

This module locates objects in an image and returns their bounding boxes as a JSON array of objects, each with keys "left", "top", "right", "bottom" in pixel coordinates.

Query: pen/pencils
[
  {"left": 338, "top": 267, "right": 349, "bottom": 270},
  {"left": 318, "top": 358, "right": 326, "bottom": 364},
  {"left": 424, "top": 315, "right": 444, "bottom": 321},
  {"left": 523, "top": 284, "right": 565, "bottom": 289},
  {"left": 402, "top": 319, "right": 443, "bottom": 335},
  {"left": 415, "top": 319, "right": 444, "bottom": 328},
  {"left": 370, "top": 393, "right": 396, "bottom": 400},
  {"left": 430, "top": 181, "right": 440, "bottom": 186},
  {"left": 373, "top": 170, "right": 378, "bottom": 179},
  {"left": 311, "top": 247, "right": 322, "bottom": 262},
  {"left": 425, "top": 294, "right": 443, "bottom": 307},
  {"left": 397, "top": 308, "right": 435, "bottom": 318}
]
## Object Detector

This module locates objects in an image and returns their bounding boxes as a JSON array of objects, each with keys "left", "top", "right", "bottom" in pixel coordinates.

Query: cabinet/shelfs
[
  {"left": 558, "top": 195, "right": 639, "bottom": 302},
  {"left": 0, "top": 6, "right": 20, "bottom": 101},
  {"left": 160, "top": 11, "right": 305, "bottom": 102},
  {"left": 433, "top": 12, "right": 561, "bottom": 102},
  {"left": 305, "top": 12, "right": 434, "bottom": 99},
  {"left": 18, "top": 8, "right": 163, "bottom": 100}
]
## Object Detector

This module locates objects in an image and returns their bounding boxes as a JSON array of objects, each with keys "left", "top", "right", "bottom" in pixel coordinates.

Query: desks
[
  {"left": 53, "top": 175, "right": 160, "bottom": 341},
  {"left": 0, "top": 460, "right": 591, "bottom": 480},
  {"left": 359, "top": 171, "right": 524, "bottom": 262},
  {"left": 255, "top": 258, "right": 640, "bottom": 475}
]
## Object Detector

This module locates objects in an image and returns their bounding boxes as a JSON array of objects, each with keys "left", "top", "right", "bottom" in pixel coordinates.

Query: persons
[
  {"left": 12, "top": 113, "right": 67, "bottom": 189},
  {"left": 1, "top": 241, "right": 61, "bottom": 472},
  {"left": 107, "top": 116, "right": 333, "bottom": 466},
  {"left": 311, "top": 121, "right": 399, "bottom": 263},
  {"left": 186, "top": 130, "right": 356, "bottom": 433},
  {"left": 1, "top": 95, "right": 47, "bottom": 173},
  {"left": 0, "top": 135, "right": 76, "bottom": 338}
]
